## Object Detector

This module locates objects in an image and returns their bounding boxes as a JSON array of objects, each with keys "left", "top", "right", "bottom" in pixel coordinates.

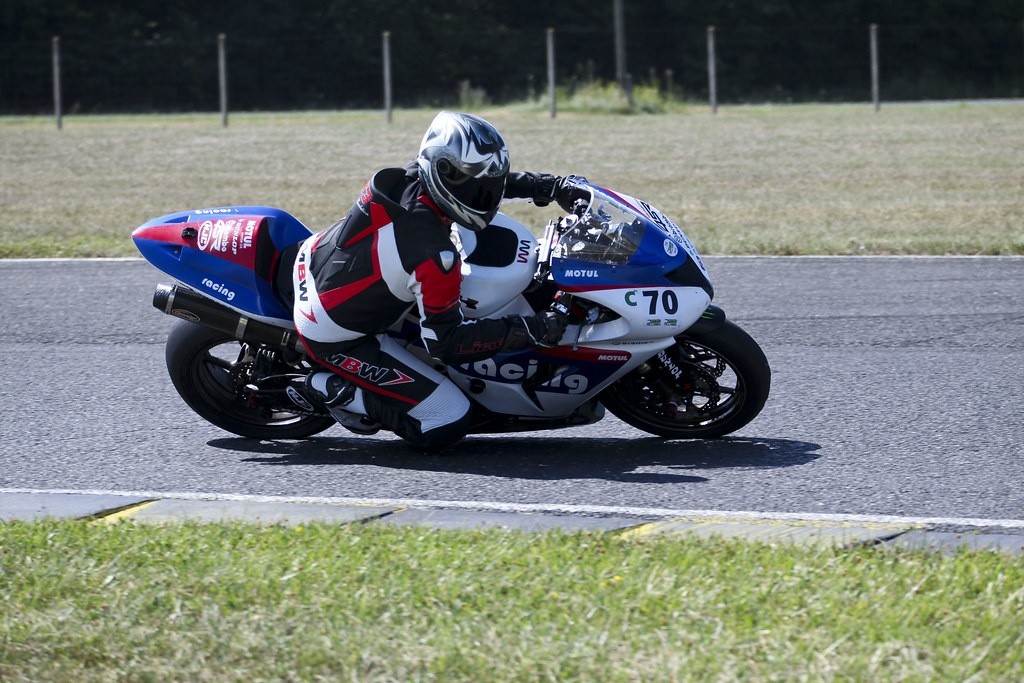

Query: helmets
[{"left": 417, "top": 111, "right": 510, "bottom": 230}]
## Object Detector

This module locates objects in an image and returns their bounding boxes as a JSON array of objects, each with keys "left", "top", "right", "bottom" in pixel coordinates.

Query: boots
[{"left": 305, "top": 373, "right": 382, "bottom": 435}]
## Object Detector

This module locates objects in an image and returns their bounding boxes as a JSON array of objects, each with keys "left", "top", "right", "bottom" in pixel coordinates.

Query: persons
[{"left": 294, "top": 114, "right": 594, "bottom": 448}]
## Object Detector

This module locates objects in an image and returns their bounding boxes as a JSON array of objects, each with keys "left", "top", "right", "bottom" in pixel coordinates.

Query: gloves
[
  {"left": 533, "top": 174, "right": 591, "bottom": 213},
  {"left": 497, "top": 310, "right": 568, "bottom": 352}
]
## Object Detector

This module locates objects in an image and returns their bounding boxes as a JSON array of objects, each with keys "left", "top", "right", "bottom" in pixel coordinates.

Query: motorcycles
[{"left": 130, "top": 174, "right": 773, "bottom": 439}]
